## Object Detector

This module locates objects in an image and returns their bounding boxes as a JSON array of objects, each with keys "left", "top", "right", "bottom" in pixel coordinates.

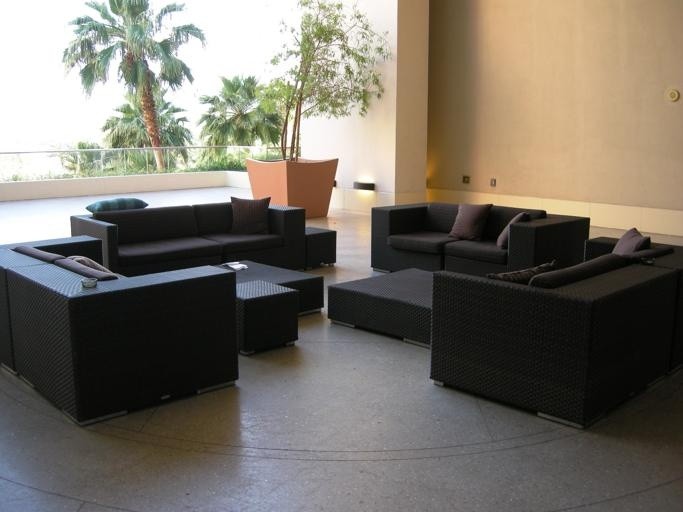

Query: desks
[
  {"left": 327, "top": 269, "right": 433, "bottom": 350},
  {"left": 222, "top": 259, "right": 324, "bottom": 317}
]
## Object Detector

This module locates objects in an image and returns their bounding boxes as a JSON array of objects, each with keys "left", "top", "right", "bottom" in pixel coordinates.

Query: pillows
[
  {"left": 447, "top": 202, "right": 492, "bottom": 240},
  {"left": 494, "top": 210, "right": 530, "bottom": 249},
  {"left": 84, "top": 197, "right": 147, "bottom": 214},
  {"left": 227, "top": 196, "right": 272, "bottom": 236},
  {"left": 69, "top": 253, "right": 111, "bottom": 275},
  {"left": 609, "top": 227, "right": 650, "bottom": 256}
]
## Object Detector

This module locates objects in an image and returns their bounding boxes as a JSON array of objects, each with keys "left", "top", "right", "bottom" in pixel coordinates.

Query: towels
[{"left": 484, "top": 257, "right": 555, "bottom": 284}]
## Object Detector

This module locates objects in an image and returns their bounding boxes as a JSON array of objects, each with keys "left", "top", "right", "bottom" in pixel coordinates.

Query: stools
[
  {"left": 230, "top": 279, "right": 299, "bottom": 357},
  {"left": 302, "top": 225, "right": 336, "bottom": 270}
]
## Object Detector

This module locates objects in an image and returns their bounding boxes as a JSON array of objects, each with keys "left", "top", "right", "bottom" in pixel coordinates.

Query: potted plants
[{"left": 242, "top": 1, "right": 390, "bottom": 220}]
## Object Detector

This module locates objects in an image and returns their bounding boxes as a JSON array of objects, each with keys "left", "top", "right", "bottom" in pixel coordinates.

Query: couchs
[
  {"left": 72, "top": 194, "right": 306, "bottom": 276},
  {"left": 369, "top": 200, "right": 589, "bottom": 276},
  {"left": 426, "top": 226, "right": 679, "bottom": 430},
  {"left": 0, "top": 231, "right": 237, "bottom": 427}
]
